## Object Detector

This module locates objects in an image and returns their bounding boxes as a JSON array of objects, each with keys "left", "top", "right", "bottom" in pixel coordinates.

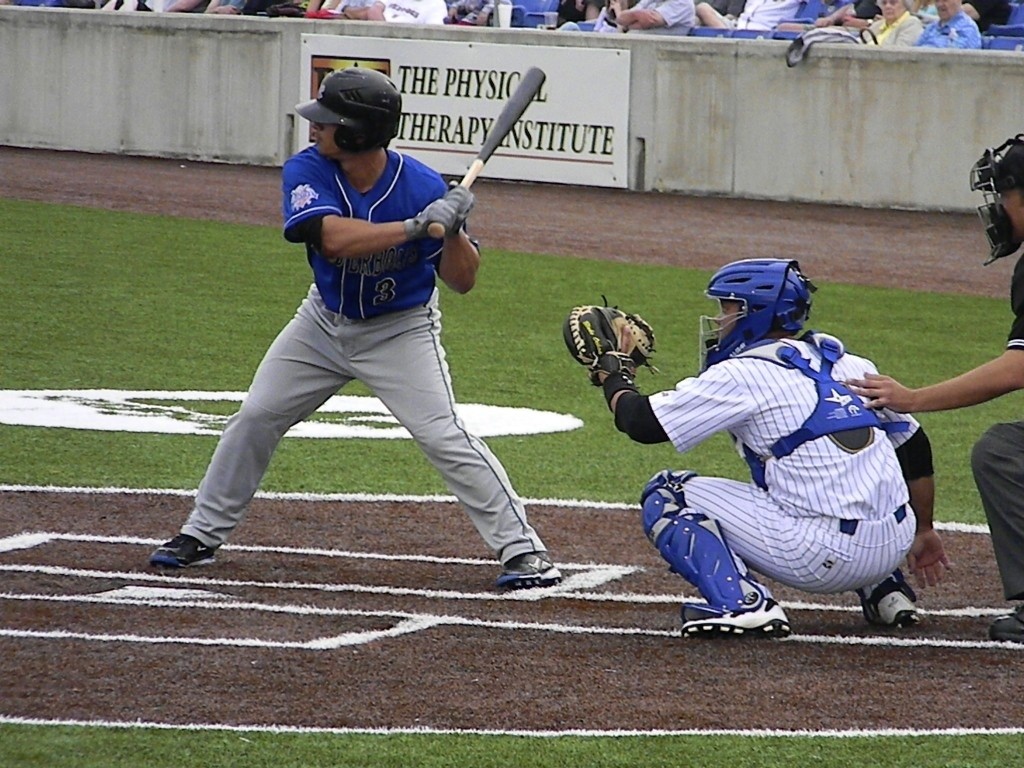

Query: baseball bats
[{"left": 427, "top": 68, "right": 546, "bottom": 238}]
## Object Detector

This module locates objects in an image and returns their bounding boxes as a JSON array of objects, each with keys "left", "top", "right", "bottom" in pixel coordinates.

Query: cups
[
  {"left": 498, "top": 5, "right": 513, "bottom": 28},
  {"left": 543, "top": 12, "right": 559, "bottom": 29}
]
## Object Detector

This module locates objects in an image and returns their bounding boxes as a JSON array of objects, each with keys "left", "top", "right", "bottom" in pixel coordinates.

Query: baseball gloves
[{"left": 562, "top": 304, "right": 656, "bottom": 385}]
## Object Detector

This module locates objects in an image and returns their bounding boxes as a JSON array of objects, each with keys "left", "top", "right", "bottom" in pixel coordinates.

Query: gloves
[
  {"left": 444, "top": 179, "right": 476, "bottom": 236},
  {"left": 403, "top": 198, "right": 460, "bottom": 245}
]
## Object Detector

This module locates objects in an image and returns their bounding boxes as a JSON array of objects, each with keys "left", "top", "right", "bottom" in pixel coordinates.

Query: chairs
[{"left": 494, "top": 0, "right": 1024, "bottom": 53}]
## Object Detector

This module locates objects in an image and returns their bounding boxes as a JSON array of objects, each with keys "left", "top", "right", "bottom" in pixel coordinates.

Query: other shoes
[{"left": 987, "top": 602, "right": 1024, "bottom": 646}]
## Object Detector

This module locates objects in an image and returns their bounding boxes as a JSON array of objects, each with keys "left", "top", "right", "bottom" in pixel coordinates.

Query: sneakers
[
  {"left": 854, "top": 568, "right": 921, "bottom": 629},
  {"left": 680, "top": 596, "right": 791, "bottom": 639},
  {"left": 493, "top": 550, "right": 564, "bottom": 591},
  {"left": 148, "top": 532, "right": 218, "bottom": 569}
]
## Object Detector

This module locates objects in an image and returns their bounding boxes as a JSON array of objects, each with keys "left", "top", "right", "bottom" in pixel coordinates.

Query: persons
[
  {"left": 148, "top": 68, "right": 564, "bottom": 592},
  {"left": 843, "top": 132, "right": 1024, "bottom": 643},
  {"left": 564, "top": 257, "right": 955, "bottom": 639},
  {"left": 18, "top": 0, "right": 1011, "bottom": 51}
]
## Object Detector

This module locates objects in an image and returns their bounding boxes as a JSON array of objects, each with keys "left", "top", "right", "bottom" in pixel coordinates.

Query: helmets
[
  {"left": 295, "top": 66, "right": 403, "bottom": 152},
  {"left": 969, "top": 130, "right": 1024, "bottom": 266},
  {"left": 697, "top": 256, "right": 819, "bottom": 374}
]
